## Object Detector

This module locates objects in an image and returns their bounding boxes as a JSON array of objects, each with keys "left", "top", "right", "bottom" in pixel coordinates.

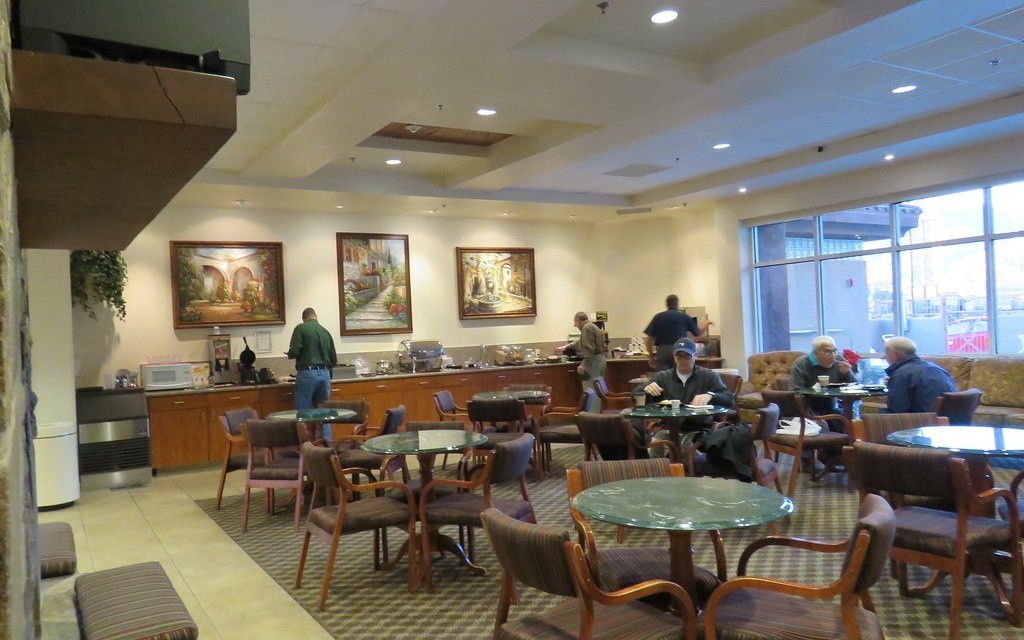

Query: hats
[{"left": 673, "top": 337, "right": 697, "bottom": 356}]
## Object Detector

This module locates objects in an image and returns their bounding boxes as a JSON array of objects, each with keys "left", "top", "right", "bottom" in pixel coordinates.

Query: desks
[
  {"left": 267, "top": 407, "right": 356, "bottom": 507},
  {"left": 886, "top": 425, "right": 1024, "bottom": 617},
  {"left": 360, "top": 430, "right": 488, "bottom": 575},
  {"left": 473, "top": 390, "right": 550, "bottom": 467},
  {"left": 618, "top": 403, "right": 727, "bottom": 464},
  {"left": 796, "top": 386, "right": 888, "bottom": 483},
  {"left": 572, "top": 476, "right": 794, "bottom": 618}
]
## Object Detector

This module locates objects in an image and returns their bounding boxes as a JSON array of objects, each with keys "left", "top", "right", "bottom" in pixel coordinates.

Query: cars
[{"left": 947, "top": 316, "right": 989, "bottom": 356}]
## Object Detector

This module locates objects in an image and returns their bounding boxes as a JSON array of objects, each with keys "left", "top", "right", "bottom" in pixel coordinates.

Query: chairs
[{"left": 215, "top": 376, "right": 1024, "bottom": 640}]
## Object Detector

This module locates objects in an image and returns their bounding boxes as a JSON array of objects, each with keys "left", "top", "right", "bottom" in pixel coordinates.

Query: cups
[
  {"left": 635, "top": 392, "right": 646, "bottom": 407},
  {"left": 629, "top": 336, "right": 646, "bottom": 352},
  {"left": 503, "top": 387, "right": 509, "bottom": 396},
  {"left": 818, "top": 376, "right": 829, "bottom": 386},
  {"left": 561, "top": 355, "right": 569, "bottom": 362},
  {"left": 671, "top": 400, "right": 680, "bottom": 408}
]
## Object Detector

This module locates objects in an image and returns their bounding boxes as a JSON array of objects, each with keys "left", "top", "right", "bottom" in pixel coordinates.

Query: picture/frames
[
  {"left": 455, "top": 246, "right": 538, "bottom": 320},
  {"left": 336, "top": 232, "right": 414, "bottom": 336},
  {"left": 169, "top": 240, "right": 286, "bottom": 328}
]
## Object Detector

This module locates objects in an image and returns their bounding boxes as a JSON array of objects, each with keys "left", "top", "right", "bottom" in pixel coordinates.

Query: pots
[{"left": 240, "top": 336, "right": 256, "bottom": 365}]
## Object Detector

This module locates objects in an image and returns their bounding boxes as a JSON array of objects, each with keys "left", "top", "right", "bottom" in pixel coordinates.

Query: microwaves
[{"left": 140, "top": 363, "right": 193, "bottom": 391}]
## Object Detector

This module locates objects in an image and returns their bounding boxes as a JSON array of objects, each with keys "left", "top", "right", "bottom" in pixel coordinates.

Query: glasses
[
  {"left": 814, "top": 349, "right": 837, "bottom": 355},
  {"left": 674, "top": 354, "right": 692, "bottom": 360}
]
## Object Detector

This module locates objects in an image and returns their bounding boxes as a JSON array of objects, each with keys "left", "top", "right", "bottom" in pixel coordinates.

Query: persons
[
  {"left": 642, "top": 294, "right": 714, "bottom": 373},
  {"left": 287, "top": 308, "right": 338, "bottom": 442},
  {"left": 788, "top": 335, "right": 861, "bottom": 472},
  {"left": 627, "top": 337, "right": 734, "bottom": 453},
  {"left": 877, "top": 336, "right": 959, "bottom": 413},
  {"left": 556, "top": 312, "right": 606, "bottom": 392}
]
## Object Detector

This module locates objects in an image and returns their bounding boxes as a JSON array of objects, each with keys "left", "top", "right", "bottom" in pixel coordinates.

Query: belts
[{"left": 302, "top": 367, "right": 325, "bottom": 370}]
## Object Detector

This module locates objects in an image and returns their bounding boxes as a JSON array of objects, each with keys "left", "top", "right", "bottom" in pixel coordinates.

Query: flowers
[{"left": 842, "top": 349, "right": 861, "bottom": 374}]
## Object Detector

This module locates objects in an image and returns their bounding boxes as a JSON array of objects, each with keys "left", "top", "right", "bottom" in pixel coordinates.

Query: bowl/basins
[
  {"left": 377, "top": 360, "right": 396, "bottom": 369},
  {"left": 547, "top": 359, "right": 559, "bottom": 363}
]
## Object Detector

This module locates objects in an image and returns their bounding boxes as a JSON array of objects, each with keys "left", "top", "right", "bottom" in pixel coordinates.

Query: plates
[
  {"left": 656, "top": 402, "right": 683, "bottom": 408},
  {"left": 821, "top": 383, "right": 845, "bottom": 386},
  {"left": 862, "top": 387, "right": 886, "bottom": 391}
]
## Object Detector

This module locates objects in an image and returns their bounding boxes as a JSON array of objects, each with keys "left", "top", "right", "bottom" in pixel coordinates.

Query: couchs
[
  {"left": 736, "top": 349, "right": 808, "bottom": 424},
  {"left": 861, "top": 354, "right": 1024, "bottom": 431}
]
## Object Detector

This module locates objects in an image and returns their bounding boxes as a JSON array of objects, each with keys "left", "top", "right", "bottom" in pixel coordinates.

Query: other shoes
[
  {"left": 802, "top": 457, "right": 813, "bottom": 473},
  {"left": 817, "top": 456, "right": 844, "bottom": 471}
]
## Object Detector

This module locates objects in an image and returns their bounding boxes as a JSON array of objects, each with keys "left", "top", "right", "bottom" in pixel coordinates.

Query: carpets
[{"left": 191, "top": 446, "right": 1024, "bottom": 640}]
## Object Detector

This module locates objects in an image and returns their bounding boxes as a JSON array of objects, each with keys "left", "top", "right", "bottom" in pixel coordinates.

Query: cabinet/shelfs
[{"left": 143, "top": 365, "right": 578, "bottom": 476}]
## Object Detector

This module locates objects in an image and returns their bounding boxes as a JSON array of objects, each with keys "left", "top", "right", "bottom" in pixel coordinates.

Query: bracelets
[{"left": 707, "top": 391, "right": 715, "bottom": 399}]
